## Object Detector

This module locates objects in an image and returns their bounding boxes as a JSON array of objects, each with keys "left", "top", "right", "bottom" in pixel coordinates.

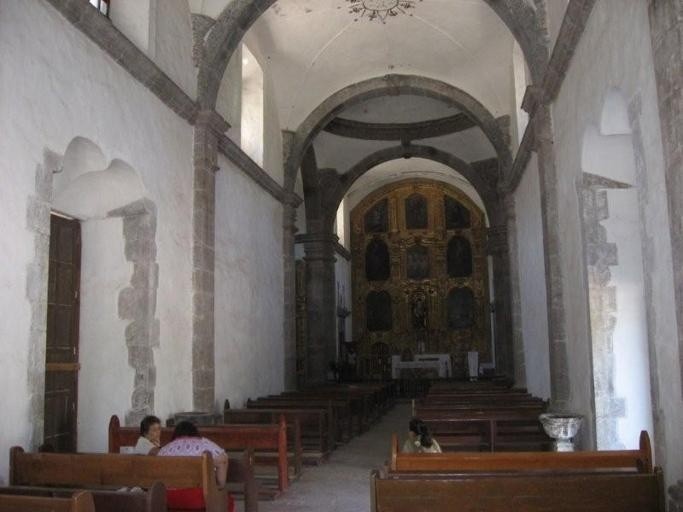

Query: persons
[
  {"left": 401, "top": 419, "right": 442, "bottom": 454},
  {"left": 155, "top": 421, "right": 235, "bottom": 511},
  {"left": 134, "top": 415, "right": 161, "bottom": 456}
]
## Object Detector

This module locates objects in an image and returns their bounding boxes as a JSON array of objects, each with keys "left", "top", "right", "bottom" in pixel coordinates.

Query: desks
[{"left": 392, "top": 354, "right": 451, "bottom": 397}]
